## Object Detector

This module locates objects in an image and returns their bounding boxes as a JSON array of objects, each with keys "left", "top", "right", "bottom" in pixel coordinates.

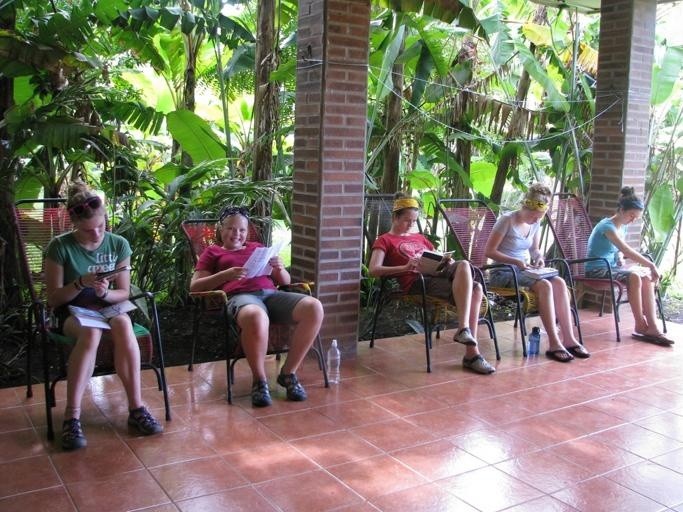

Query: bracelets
[
  {"left": 73, "top": 275, "right": 86, "bottom": 291},
  {"left": 97, "top": 289, "right": 109, "bottom": 299}
]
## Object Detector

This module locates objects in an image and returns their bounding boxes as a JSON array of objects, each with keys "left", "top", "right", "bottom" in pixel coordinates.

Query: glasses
[{"left": 67, "top": 196, "right": 102, "bottom": 218}]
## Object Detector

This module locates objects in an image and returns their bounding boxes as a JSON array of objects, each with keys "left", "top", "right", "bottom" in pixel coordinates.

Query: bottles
[
  {"left": 526, "top": 327, "right": 540, "bottom": 355},
  {"left": 326, "top": 340, "right": 340, "bottom": 384}
]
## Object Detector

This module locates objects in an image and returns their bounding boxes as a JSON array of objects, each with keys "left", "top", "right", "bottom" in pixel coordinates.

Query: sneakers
[
  {"left": 60, "top": 418, "right": 88, "bottom": 449},
  {"left": 462, "top": 353, "right": 496, "bottom": 375},
  {"left": 127, "top": 405, "right": 164, "bottom": 435},
  {"left": 251, "top": 376, "right": 273, "bottom": 407},
  {"left": 276, "top": 368, "right": 306, "bottom": 401},
  {"left": 454, "top": 327, "right": 477, "bottom": 346}
]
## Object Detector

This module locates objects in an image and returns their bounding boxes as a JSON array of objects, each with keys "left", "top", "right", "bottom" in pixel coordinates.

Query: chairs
[
  {"left": 437, "top": 190, "right": 583, "bottom": 368},
  {"left": 11, "top": 194, "right": 171, "bottom": 441},
  {"left": 545, "top": 192, "right": 668, "bottom": 342}
]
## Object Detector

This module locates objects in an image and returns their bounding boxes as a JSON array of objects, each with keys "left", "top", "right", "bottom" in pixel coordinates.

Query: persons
[
  {"left": 484, "top": 183, "right": 590, "bottom": 362},
  {"left": 41, "top": 182, "right": 162, "bottom": 450},
  {"left": 585, "top": 186, "right": 674, "bottom": 347}
]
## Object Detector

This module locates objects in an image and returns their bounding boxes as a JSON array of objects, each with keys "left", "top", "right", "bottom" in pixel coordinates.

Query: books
[
  {"left": 64, "top": 299, "right": 140, "bottom": 329},
  {"left": 523, "top": 265, "right": 561, "bottom": 278},
  {"left": 416, "top": 249, "right": 458, "bottom": 280},
  {"left": 95, "top": 264, "right": 132, "bottom": 282},
  {"left": 238, "top": 241, "right": 280, "bottom": 280},
  {"left": 611, "top": 265, "right": 653, "bottom": 278}
]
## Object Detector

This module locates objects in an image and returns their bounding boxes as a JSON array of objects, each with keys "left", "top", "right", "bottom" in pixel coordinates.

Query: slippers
[
  {"left": 545, "top": 349, "right": 574, "bottom": 362},
  {"left": 631, "top": 330, "right": 675, "bottom": 345},
  {"left": 567, "top": 345, "right": 591, "bottom": 358}
]
